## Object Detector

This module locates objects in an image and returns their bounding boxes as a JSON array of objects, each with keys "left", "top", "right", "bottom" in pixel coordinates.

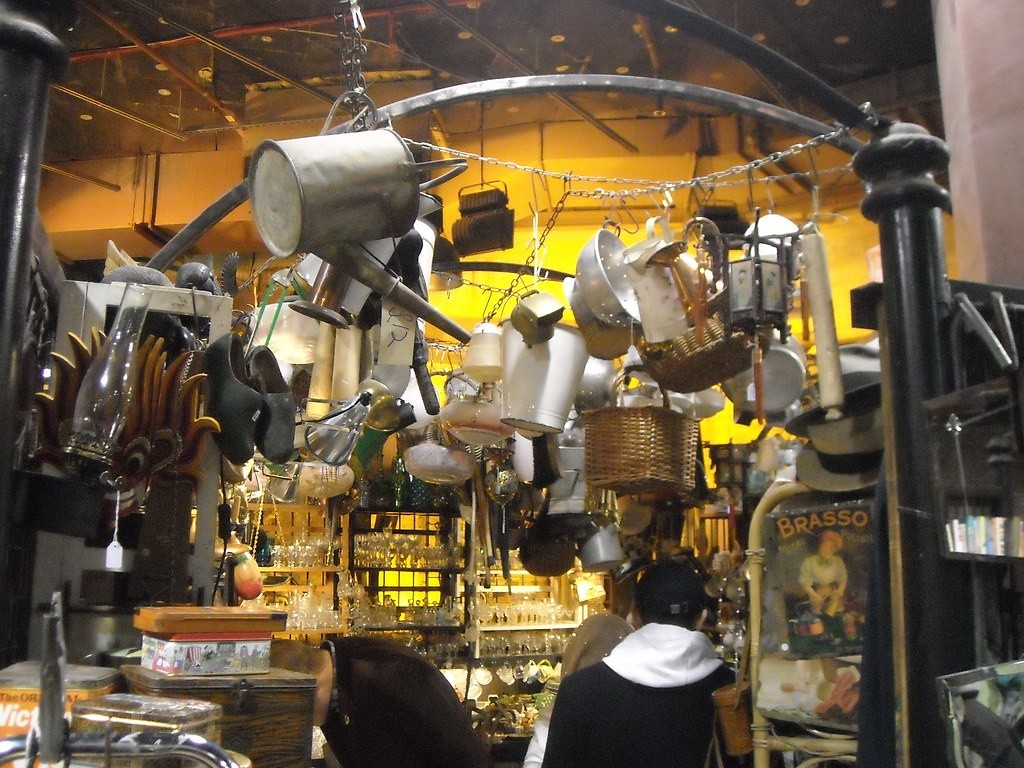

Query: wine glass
[
  {"left": 555, "top": 604, "right": 566, "bottom": 625},
  {"left": 353, "top": 527, "right": 463, "bottom": 569},
  {"left": 565, "top": 609, "right": 575, "bottom": 623},
  {"left": 316, "top": 537, "right": 331, "bottom": 568}
]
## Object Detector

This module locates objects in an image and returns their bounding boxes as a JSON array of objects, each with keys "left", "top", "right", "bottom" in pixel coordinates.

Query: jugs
[
  {"left": 622, "top": 215, "right": 726, "bottom": 344},
  {"left": 247, "top": 127, "right": 470, "bottom": 350}
]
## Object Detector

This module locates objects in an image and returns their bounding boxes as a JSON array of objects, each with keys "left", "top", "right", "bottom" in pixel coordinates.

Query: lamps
[{"left": 429, "top": 180, "right": 514, "bottom": 292}]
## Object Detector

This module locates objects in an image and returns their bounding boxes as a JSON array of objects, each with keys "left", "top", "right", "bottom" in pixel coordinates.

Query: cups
[
  {"left": 495, "top": 659, "right": 553, "bottom": 732},
  {"left": 460, "top": 323, "right": 502, "bottom": 383},
  {"left": 480, "top": 633, "right": 568, "bottom": 656},
  {"left": 458, "top": 663, "right": 493, "bottom": 700},
  {"left": 268, "top": 545, "right": 316, "bottom": 569},
  {"left": 479, "top": 602, "right": 557, "bottom": 627},
  {"left": 410, "top": 642, "right": 460, "bottom": 659}
]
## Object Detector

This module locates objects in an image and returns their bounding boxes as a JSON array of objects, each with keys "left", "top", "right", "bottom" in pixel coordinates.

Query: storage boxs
[{"left": 0, "top": 604, "right": 318, "bottom": 768}]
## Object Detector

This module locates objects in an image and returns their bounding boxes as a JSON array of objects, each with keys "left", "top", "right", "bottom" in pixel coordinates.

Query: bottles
[
  {"left": 403, "top": 596, "right": 463, "bottom": 624},
  {"left": 58, "top": 280, "right": 153, "bottom": 467}
]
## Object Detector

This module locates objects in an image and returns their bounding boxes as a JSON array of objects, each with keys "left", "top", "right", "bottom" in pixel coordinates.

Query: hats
[{"left": 635, "top": 560, "right": 705, "bottom": 615}]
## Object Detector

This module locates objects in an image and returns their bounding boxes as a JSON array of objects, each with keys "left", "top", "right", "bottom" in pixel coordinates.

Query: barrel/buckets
[
  {"left": 341, "top": 216, "right": 438, "bottom": 337},
  {"left": 547, "top": 445, "right": 586, "bottom": 515},
  {"left": 722, "top": 331, "right": 808, "bottom": 413},
  {"left": 498, "top": 317, "right": 590, "bottom": 434}
]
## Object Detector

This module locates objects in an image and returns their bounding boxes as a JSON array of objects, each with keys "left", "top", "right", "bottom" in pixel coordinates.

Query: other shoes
[
  {"left": 245, "top": 345, "right": 297, "bottom": 466},
  {"left": 205, "top": 331, "right": 263, "bottom": 465}
]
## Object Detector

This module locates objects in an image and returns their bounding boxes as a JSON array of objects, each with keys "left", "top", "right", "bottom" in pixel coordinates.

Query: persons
[
  {"left": 538, "top": 559, "right": 785, "bottom": 767},
  {"left": 798, "top": 527, "right": 865, "bottom": 658},
  {"left": 523, "top": 612, "right": 639, "bottom": 768},
  {"left": 266, "top": 636, "right": 491, "bottom": 768}
]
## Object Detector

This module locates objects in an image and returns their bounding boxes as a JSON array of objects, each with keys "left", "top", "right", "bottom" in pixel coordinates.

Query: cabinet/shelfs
[{"left": 192, "top": 502, "right": 608, "bottom": 660}]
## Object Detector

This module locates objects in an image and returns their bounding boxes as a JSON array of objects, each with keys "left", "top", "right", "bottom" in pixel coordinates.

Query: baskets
[
  {"left": 637, "top": 306, "right": 775, "bottom": 395},
  {"left": 582, "top": 363, "right": 702, "bottom": 494}
]
